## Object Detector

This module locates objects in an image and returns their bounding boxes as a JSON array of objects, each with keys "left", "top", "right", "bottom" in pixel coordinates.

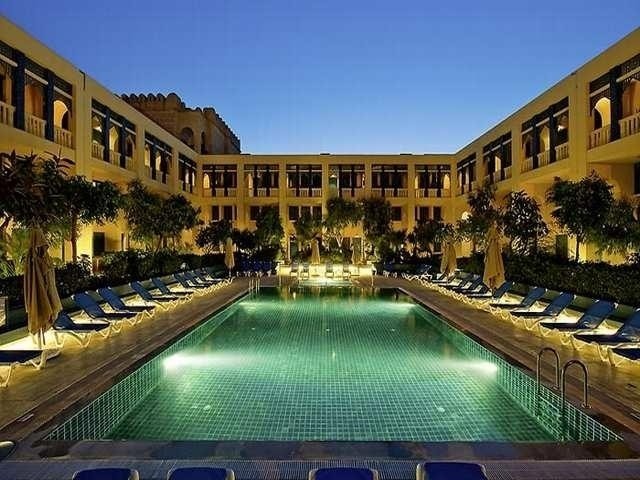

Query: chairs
[
  {"left": 0, "top": 259, "right": 273, "bottom": 374},
  {"left": 383, "top": 264, "right": 640, "bottom": 368},
  {"left": 290, "top": 259, "right": 351, "bottom": 281}
]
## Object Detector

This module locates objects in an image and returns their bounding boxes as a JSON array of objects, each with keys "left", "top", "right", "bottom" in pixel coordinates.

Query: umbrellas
[
  {"left": 310, "top": 239, "right": 321, "bottom": 273},
  {"left": 352, "top": 240, "right": 363, "bottom": 274},
  {"left": 439, "top": 236, "right": 458, "bottom": 283},
  {"left": 24, "top": 217, "right": 63, "bottom": 347},
  {"left": 224, "top": 237, "right": 236, "bottom": 277},
  {"left": 481, "top": 225, "right": 507, "bottom": 304}
]
